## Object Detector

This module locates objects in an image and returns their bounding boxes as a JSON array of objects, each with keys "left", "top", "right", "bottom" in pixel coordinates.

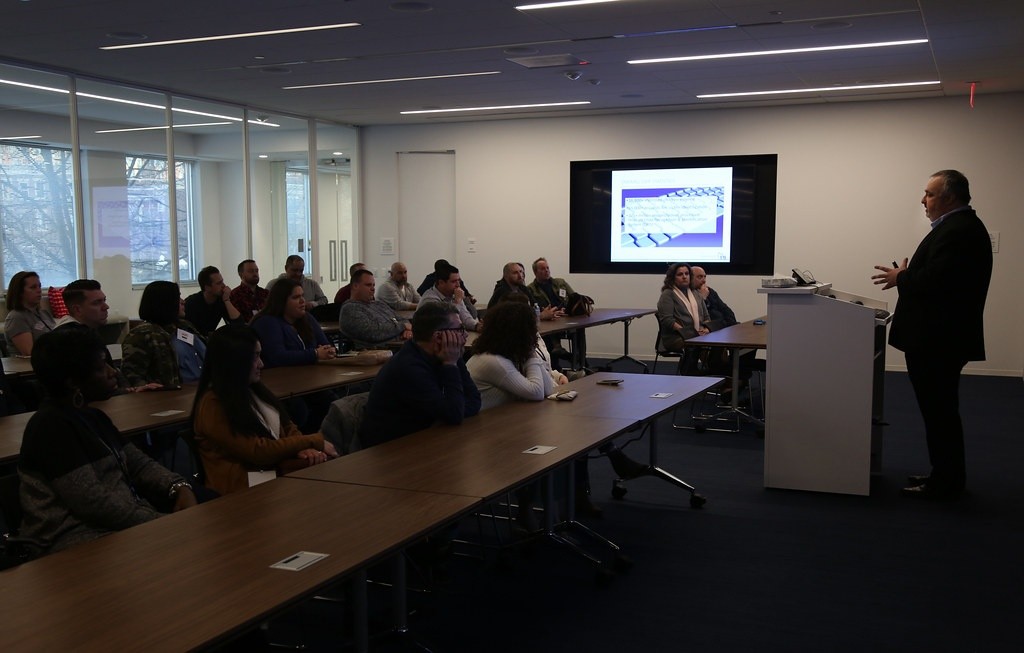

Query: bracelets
[
  {"left": 169, "top": 482, "right": 193, "bottom": 497},
  {"left": 224, "top": 299, "right": 230, "bottom": 301}
]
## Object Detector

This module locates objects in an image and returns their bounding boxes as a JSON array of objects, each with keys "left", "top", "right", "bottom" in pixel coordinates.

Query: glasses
[{"left": 434, "top": 324, "right": 465, "bottom": 338}]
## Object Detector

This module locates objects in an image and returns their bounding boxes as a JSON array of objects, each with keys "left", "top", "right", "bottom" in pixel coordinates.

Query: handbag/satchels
[
  {"left": 661, "top": 330, "right": 684, "bottom": 351},
  {"left": 557, "top": 292, "right": 595, "bottom": 318},
  {"left": 49, "top": 287, "right": 70, "bottom": 319},
  {"left": 318, "top": 349, "right": 394, "bottom": 366}
]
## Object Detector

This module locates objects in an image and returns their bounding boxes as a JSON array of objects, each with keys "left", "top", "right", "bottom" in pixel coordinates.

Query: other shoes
[
  {"left": 519, "top": 503, "right": 540, "bottom": 532},
  {"left": 720, "top": 387, "right": 734, "bottom": 402}
]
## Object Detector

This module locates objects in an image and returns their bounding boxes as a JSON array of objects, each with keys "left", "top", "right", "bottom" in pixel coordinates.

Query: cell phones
[
  {"left": 596, "top": 379, "right": 624, "bottom": 384},
  {"left": 337, "top": 353, "right": 358, "bottom": 358}
]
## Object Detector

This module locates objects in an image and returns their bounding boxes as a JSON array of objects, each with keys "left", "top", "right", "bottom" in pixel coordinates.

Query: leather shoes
[
  {"left": 907, "top": 473, "right": 929, "bottom": 486},
  {"left": 901, "top": 483, "right": 946, "bottom": 500}
]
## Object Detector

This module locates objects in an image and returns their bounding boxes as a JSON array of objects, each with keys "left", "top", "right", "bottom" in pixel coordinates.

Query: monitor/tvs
[{"left": 792, "top": 268, "right": 812, "bottom": 283}]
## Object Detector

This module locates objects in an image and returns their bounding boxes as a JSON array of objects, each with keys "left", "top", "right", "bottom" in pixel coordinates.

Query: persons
[
  {"left": 417, "top": 259, "right": 472, "bottom": 296},
  {"left": 357, "top": 300, "right": 482, "bottom": 449},
  {"left": 334, "top": 263, "right": 367, "bottom": 302},
  {"left": 249, "top": 276, "right": 337, "bottom": 367},
  {"left": 16, "top": 322, "right": 197, "bottom": 558},
  {"left": 871, "top": 170, "right": 993, "bottom": 501},
  {"left": 191, "top": 323, "right": 340, "bottom": 496},
  {"left": 417, "top": 265, "right": 484, "bottom": 332},
  {"left": 657, "top": 263, "right": 756, "bottom": 393},
  {"left": 265, "top": 255, "right": 328, "bottom": 309},
  {"left": 376, "top": 262, "right": 421, "bottom": 310},
  {"left": 184, "top": 266, "right": 245, "bottom": 334},
  {"left": 339, "top": 269, "right": 413, "bottom": 354},
  {"left": 467, "top": 291, "right": 651, "bottom": 530},
  {"left": 3, "top": 271, "right": 57, "bottom": 355},
  {"left": 232, "top": 260, "right": 277, "bottom": 322},
  {"left": 488, "top": 257, "right": 575, "bottom": 349},
  {"left": 53, "top": 279, "right": 164, "bottom": 393},
  {"left": 121, "top": 281, "right": 208, "bottom": 388}
]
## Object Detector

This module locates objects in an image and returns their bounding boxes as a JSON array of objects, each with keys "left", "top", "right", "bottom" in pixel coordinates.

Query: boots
[
  {"left": 574, "top": 483, "right": 605, "bottom": 516},
  {"left": 607, "top": 448, "right": 652, "bottom": 480}
]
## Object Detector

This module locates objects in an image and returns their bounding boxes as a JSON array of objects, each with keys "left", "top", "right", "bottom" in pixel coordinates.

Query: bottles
[{"left": 532, "top": 303, "right": 540, "bottom": 326}]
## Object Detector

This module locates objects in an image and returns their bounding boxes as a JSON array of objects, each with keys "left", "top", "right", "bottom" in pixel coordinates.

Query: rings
[
  {"left": 318, "top": 452, "right": 321, "bottom": 454},
  {"left": 410, "top": 335, "right": 411, "bottom": 336}
]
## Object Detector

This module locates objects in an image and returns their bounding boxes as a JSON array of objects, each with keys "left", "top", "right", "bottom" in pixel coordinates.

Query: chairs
[
  {"left": 322, "top": 393, "right": 365, "bottom": 453},
  {"left": 653, "top": 313, "right": 710, "bottom": 374}
]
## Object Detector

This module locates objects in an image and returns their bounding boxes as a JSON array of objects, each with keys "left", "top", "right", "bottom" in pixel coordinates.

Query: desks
[{"left": 0, "top": 308, "right": 768, "bottom": 653}]
